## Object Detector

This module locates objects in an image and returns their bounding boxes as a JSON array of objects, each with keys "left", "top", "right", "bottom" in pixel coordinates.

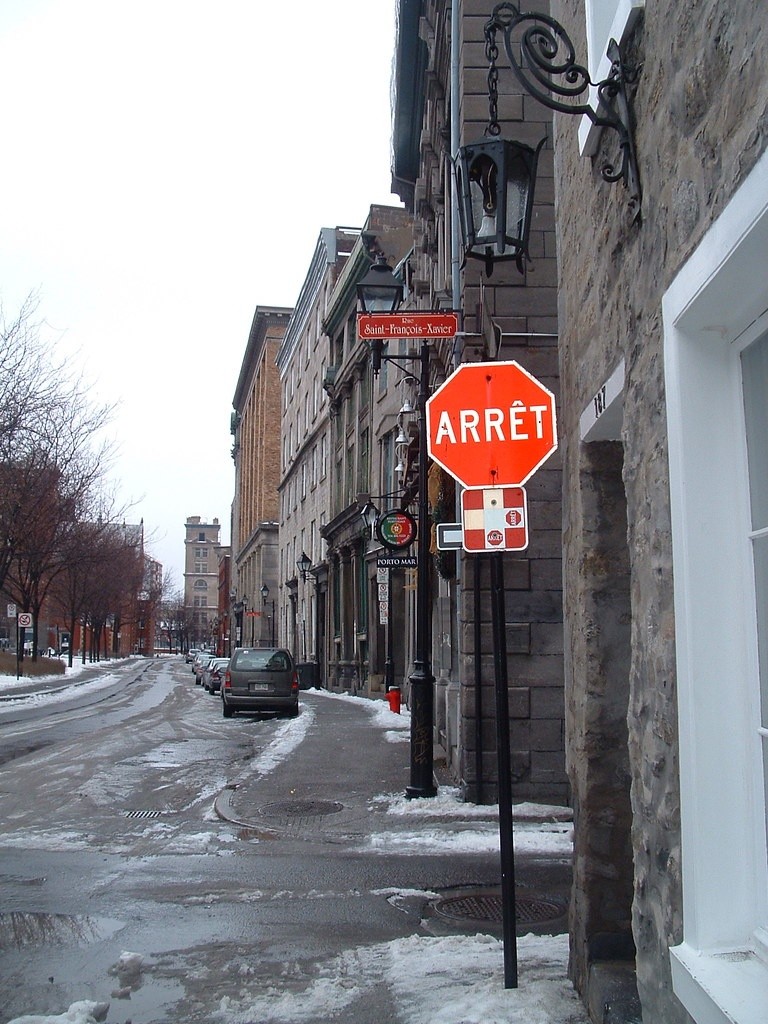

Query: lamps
[
  {"left": 453, "top": 4, "right": 642, "bottom": 279},
  {"left": 395, "top": 376, "right": 419, "bottom": 472}
]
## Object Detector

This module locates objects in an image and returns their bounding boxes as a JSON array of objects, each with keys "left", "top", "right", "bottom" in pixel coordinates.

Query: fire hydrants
[{"left": 383, "top": 685, "right": 403, "bottom": 716}]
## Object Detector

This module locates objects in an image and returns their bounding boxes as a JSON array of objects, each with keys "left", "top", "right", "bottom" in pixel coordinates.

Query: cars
[
  {"left": 191, "top": 651, "right": 240, "bottom": 697},
  {"left": 186, "top": 648, "right": 200, "bottom": 662}
]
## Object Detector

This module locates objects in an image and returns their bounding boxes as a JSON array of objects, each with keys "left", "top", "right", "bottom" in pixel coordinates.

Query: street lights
[
  {"left": 240, "top": 594, "right": 254, "bottom": 648},
  {"left": 259, "top": 581, "right": 276, "bottom": 650},
  {"left": 297, "top": 551, "right": 323, "bottom": 691},
  {"left": 354, "top": 257, "right": 437, "bottom": 800}
]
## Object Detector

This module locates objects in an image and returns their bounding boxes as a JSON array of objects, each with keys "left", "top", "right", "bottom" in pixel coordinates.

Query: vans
[{"left": 223, "top": 647, "right": 298, "bottom": 717}]
